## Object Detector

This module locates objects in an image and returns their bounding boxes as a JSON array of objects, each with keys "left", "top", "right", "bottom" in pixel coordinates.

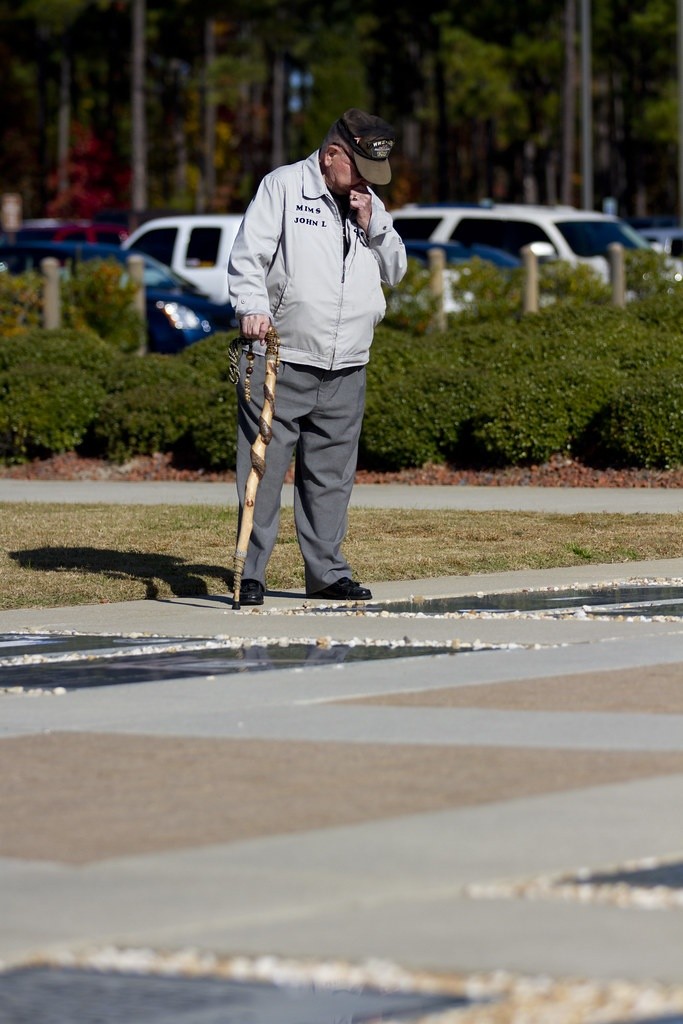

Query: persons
[{"left": 226, "top": 109, "right": 410, "bottom": 603}]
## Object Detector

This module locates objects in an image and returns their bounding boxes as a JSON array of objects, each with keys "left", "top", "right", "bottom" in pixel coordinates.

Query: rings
[{"left": 353, "top": 195, "right": 358, "bottom": 201}]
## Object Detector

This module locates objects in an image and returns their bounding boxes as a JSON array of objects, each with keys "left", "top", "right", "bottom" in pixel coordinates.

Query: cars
[
  {"left": 627, "top": 215, "right": 682, "bottom": 277},
  {"left": 0, "top": 214, "right": 556, "bottom": 356}
]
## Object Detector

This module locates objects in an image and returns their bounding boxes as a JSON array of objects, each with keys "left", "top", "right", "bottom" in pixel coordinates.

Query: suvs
[{"left": 391, "top": 201, "right": 671, "bottom": 307}]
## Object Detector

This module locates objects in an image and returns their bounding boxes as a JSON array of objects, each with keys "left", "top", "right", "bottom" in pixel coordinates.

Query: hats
[{"left": 336, "top": 108, "right": 394, "bottom": 185}]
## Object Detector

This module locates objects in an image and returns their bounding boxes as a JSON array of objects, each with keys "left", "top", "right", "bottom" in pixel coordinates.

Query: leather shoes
[
  {"left": 238, "top": 578, "right": 264, "bottom": 605},
  {"left": 306, "top": 577, "right": 373, "bottom": 600}
]
